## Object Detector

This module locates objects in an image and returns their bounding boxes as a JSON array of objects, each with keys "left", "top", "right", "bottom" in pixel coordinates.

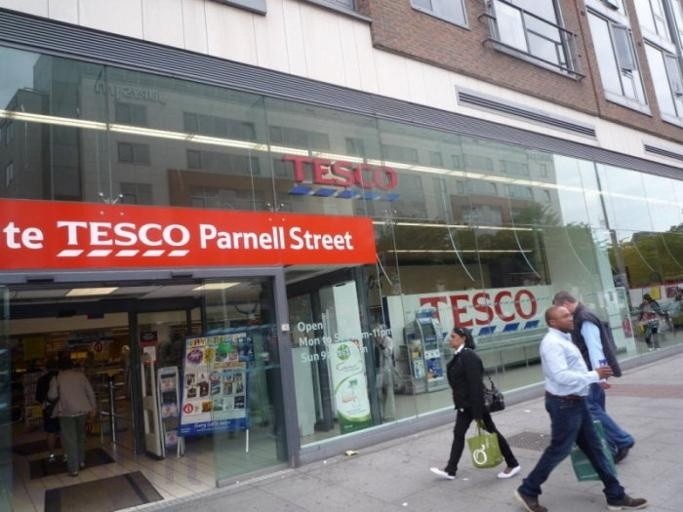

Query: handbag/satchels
[
  {"left": 569, "top": 419, "right": 618, "bottom": 480},
  {"left": 468, "top": 433, "right": 503, "bottom": 468},
  {"left": 484, "top": 386, "right": 505, "bottom": 412},
  {"left": 44, "top": 404, "right": 61, "bottom": 432}
]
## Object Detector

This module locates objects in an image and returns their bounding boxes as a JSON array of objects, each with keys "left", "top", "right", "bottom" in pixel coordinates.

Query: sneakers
[
  {"left": 517, "top": 488, "right": 547, "bottom": 512},
  {"left": 608, "top": 493, "right": 647, "bottom": 510}
]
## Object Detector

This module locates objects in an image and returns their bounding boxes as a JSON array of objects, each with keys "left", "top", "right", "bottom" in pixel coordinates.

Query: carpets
[
  {"left": 43, "top": 471, "right": 164, "bottom": 512},
  {"left": 28, "top": 445, "right": 115, "bottom": 480},
  {"left": 1, "top": 434, "right": 65, "bottom": 457}
]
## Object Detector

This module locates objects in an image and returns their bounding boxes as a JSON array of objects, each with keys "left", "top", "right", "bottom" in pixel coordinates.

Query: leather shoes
[
  {"left": 497, "top": 465, "right": 520, "bottom": 479},
  {"left": 615, "top": 443, "right": 633, "bottom": 462},
  {"left": 430, "top": 466, "right": 454, "bottom": 479}
]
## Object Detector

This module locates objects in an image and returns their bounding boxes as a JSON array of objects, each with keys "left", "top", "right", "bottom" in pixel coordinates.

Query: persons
[
  {"left": 516, "top": 304, "right": 647, "bottom": 511},
  {"left": 47, "top": 358, "right": 97, "bottom": 479},
  {"left": 372, "top": 324, "right": 398, "bottom": 419},
  {"left": 638, "top": 294, "right": 673, "bottom": 350},
  {"left": 552, "top": 290, "right": 634, "bottom": 466},
  {"left": 427, "top": 326, "right": 521, "bottom": 481}
]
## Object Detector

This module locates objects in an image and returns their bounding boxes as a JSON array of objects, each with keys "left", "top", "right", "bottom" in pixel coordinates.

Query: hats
[{"left": 455, "top": 326, "right": 475, "bottom": 349}]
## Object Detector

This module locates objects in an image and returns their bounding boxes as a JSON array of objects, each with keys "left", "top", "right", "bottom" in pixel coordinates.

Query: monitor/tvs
[{"left": 421, "top": 323, "right": 435, "bottom": 337}]
